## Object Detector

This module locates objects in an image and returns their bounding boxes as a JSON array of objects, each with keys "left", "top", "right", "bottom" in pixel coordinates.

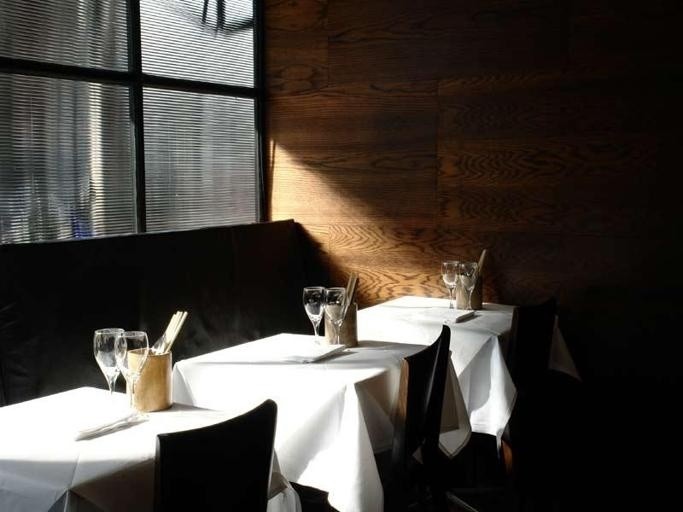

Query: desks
[
  {"left": 1, "top": 385, "right": 235, "bottom": 510},
  {"left": 356, "top": 296, "right": 518, "bottom": 436},
  {"left": 172, "top": 333, "right": 473, "bottom": 510}
]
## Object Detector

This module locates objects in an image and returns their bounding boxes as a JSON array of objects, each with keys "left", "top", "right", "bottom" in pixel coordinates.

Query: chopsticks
[
  {"left": 151, "top": 310, "right": 189, "bottom": 355},
  {"left": 341, "top": 274, "right": 359, "bottom": 307}
]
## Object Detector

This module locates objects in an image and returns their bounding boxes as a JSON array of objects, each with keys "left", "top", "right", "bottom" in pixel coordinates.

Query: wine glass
[
  {"left": 442, "top": 259, "right": 480, "bottom": 310},
  {"left": 92, "top": 328, "right": 149, "bottom": 409},
  {"left": 302, "top": 286, "right": 346, "bottom": 344}
]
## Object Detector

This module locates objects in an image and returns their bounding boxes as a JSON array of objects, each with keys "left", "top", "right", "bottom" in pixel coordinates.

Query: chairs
[
  {"left": 294, "top": 321, "right": 453, "bottom": 508},
  {"left": 69, "top": 399, "right": 276, "bottom": 511},
  {"left": 504, "top": 293, "right": 558, "bottom": 440}
]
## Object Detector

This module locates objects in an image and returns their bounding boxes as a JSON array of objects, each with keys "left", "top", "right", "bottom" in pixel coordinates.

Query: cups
[
  {"left": 456, "top": 273, "right": 482, "bottom": 310},
  {"left": 128, "top": 348, "right": 173, "bottom": 410},
  {"left": 324, "top": 303, "right": 358, "bottom": 349}
]
